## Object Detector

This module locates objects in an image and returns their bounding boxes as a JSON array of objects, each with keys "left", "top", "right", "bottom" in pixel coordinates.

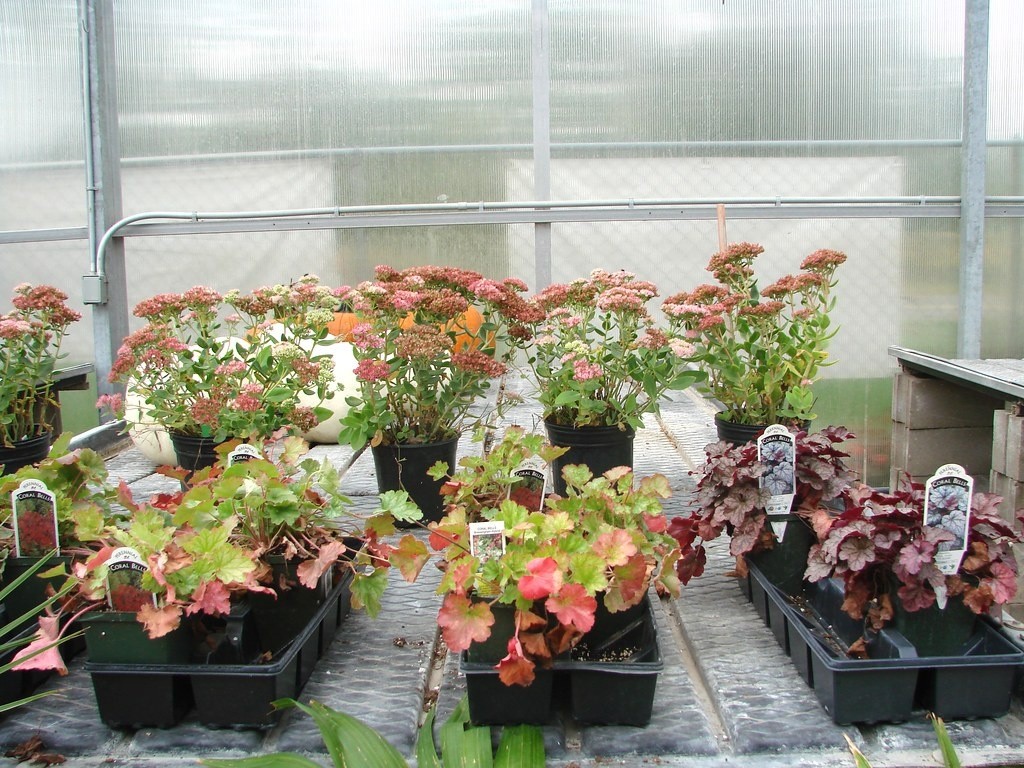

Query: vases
[
  {"left": 715, "top": 408, "right": 812, "bottom": 451},
  {"left": 0, "top": 422, "right": 53, "bottom": 476},
  {"left": 170, "top": 424, "right": 250, "bottom": 493},
  {"left": 372, "top": 425, "right": 460, "bottom": 529},
  {"left": 544, "top": 408, "right": 636, "bottom": 500}
]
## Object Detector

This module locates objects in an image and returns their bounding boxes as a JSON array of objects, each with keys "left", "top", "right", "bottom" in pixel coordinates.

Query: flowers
[
  {"left": 525, "top": 267, "right": 709, "bottom": 430},
  {"left": 335, "top": 265, "right": 511, "bottom": 450},
  {"left": 664, "top": 242, "right": 846, "bottom": 427},
  {"left": 94, "top": 274, "right": 340, "bottom": 439},
  {"left": 0, "top": 283, "right": 84, "bottom": 444}
]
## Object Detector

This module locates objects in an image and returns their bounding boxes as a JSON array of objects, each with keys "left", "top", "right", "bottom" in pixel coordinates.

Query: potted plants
[{"left": 0, "top": 436, "right": 1024, "bottom": 731}]
